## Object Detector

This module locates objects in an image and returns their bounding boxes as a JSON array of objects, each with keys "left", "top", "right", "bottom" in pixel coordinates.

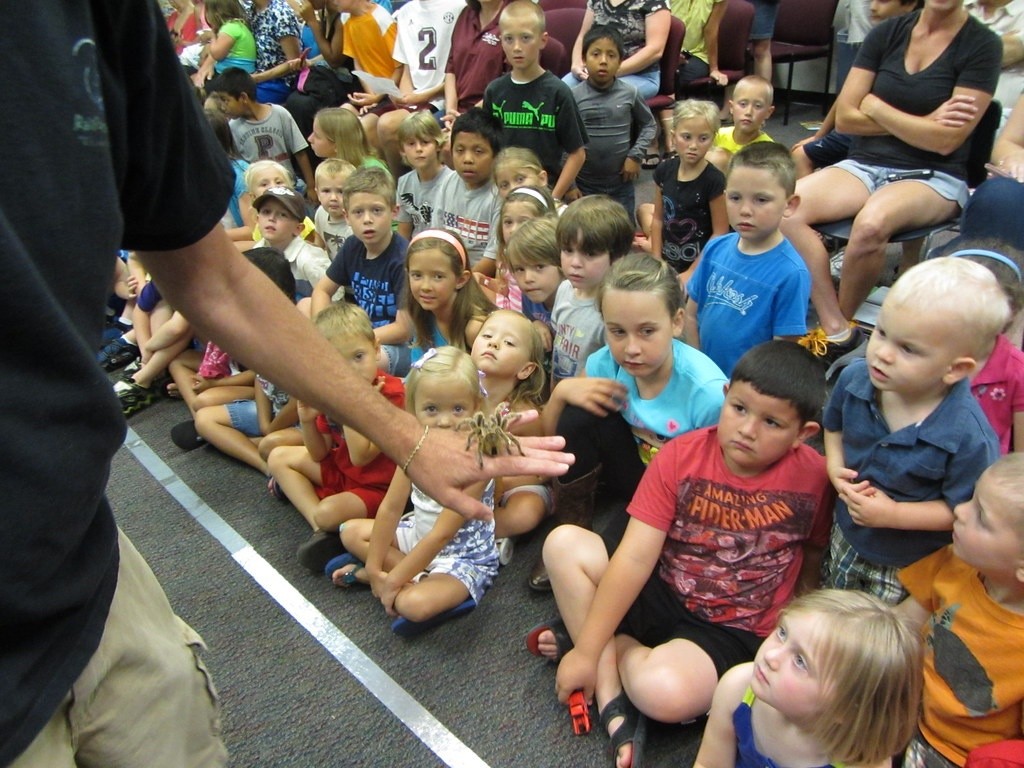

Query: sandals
[
  {"left": 525, "top": 618, "right": 575, "bottom": 663},
  {"left": 598, "top": 688, "right": 648, "bottom": 768}
]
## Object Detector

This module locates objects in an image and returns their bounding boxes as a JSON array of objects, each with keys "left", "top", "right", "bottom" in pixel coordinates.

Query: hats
[{"left": 252, "top": 186, "right": 306, "bottom": 223}]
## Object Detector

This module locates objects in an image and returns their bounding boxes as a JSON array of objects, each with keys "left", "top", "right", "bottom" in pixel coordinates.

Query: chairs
[{"left": 538, "top": 0, "right": 1001, "bottom": 264}]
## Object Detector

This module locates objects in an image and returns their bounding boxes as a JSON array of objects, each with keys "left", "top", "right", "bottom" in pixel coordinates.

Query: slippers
[
  {"left": 325, "top": 552, "right": 365, "bottom": 588},
  {"left": 391, "top": 590, "right": 476, "bottom": 635}
]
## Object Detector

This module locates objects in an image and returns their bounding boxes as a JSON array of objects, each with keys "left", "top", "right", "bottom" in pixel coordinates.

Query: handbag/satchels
[{"left": 289, "top": 62, "right": 347, "bottom": 112}]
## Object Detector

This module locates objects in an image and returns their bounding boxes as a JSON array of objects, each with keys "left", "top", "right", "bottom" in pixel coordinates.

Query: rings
[{"left": 299, "top": 3, "right": 302, "bottom": 7}]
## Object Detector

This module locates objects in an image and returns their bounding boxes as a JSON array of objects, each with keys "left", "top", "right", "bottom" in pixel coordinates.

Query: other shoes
[
  {"left": 102, "top": 315, "right": 134, "bottom": 339},
  {"left": 272, "top": 477, "right": 287, "bottom": 499},
  {"left": 296, "top": 528, "right": 345, "bottom": 574},
  {"left": 172, "top": 419, "right": 208, "bottom": 450}
]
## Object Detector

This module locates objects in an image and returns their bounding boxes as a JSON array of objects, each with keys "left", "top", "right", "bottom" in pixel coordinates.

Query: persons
[
  {"left": 95, "top": 0, "right": 1024, "bottom": 768},
  {"left": 0, "top": 0, "right": 574, "bottom": 768}
]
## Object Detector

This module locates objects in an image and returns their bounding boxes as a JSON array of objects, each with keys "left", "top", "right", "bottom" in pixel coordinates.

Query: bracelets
[{"left": 403, "top": 425, "right": 429, "bottom": 473}]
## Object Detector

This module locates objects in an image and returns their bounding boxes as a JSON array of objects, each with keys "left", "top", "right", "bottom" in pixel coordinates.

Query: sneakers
[
  {"left": 796, "top": 319, "right": 867, "bottom": 380},
  {"left": 105, "top": 373, "right": 157, "bottom": 416},
  {"left": 125, "top": 356, "right": 167, "bottom": 387},
  {"left": 95, "top": 335, "right": 142, "bottom": 373}
]
